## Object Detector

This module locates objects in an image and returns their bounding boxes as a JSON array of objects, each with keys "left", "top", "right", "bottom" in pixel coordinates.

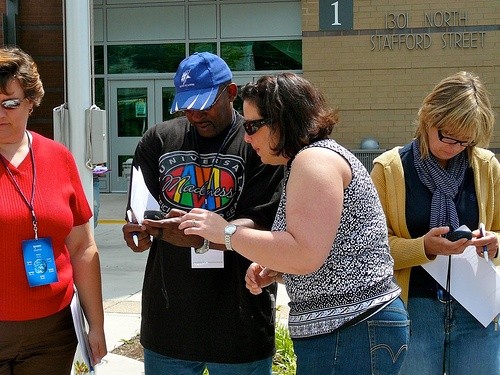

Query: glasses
[
  {"left": 243, "top": 118, "right": 268, "bottom": 135},
  {"left": 0, "top": 93, "right": 30, "bottom": 110},
  {"left": 182, "top": 83, "right": 232, "bottom": 113},
  {"left": 438, "top": 127, "right": 478, "bottom": 147}
]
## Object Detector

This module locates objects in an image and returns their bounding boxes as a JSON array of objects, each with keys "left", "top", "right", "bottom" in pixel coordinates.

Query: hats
[{"left": 170, "top": 51, "right": 232, "bottom": 114}]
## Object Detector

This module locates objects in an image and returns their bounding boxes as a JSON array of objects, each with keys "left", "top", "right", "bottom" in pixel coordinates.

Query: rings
[{"left": 191, "top": 219, "right": 195, "bottom": 227}]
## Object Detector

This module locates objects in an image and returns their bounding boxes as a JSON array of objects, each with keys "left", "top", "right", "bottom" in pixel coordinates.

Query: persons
[
  {"left": 0, "top": 46, "right": 107, "bottom": 375},
  {"left": 372, "top": 71, "right": 500, "bottom": 374},
  {"left": 178, "top": 73, "right": 411, "bottom": 374},
  {"left": 122, "top": 51, "right": 285, "bottom": 374}
]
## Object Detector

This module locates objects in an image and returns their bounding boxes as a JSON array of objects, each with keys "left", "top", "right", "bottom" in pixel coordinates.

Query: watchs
[
  {"left": 224, "top": 223, "right": 237, "bottom": 251},
  {"left": 194, "top": 239, "right": 210, "bottom": 254}
]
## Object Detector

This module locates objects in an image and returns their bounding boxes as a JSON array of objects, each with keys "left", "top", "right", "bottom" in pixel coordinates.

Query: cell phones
[
  {"left": 143, "top": 211, "right": 165, "bottom": 222},
  {"left": 447, "top": 231, "right": 472, "bottom": 242}
]
